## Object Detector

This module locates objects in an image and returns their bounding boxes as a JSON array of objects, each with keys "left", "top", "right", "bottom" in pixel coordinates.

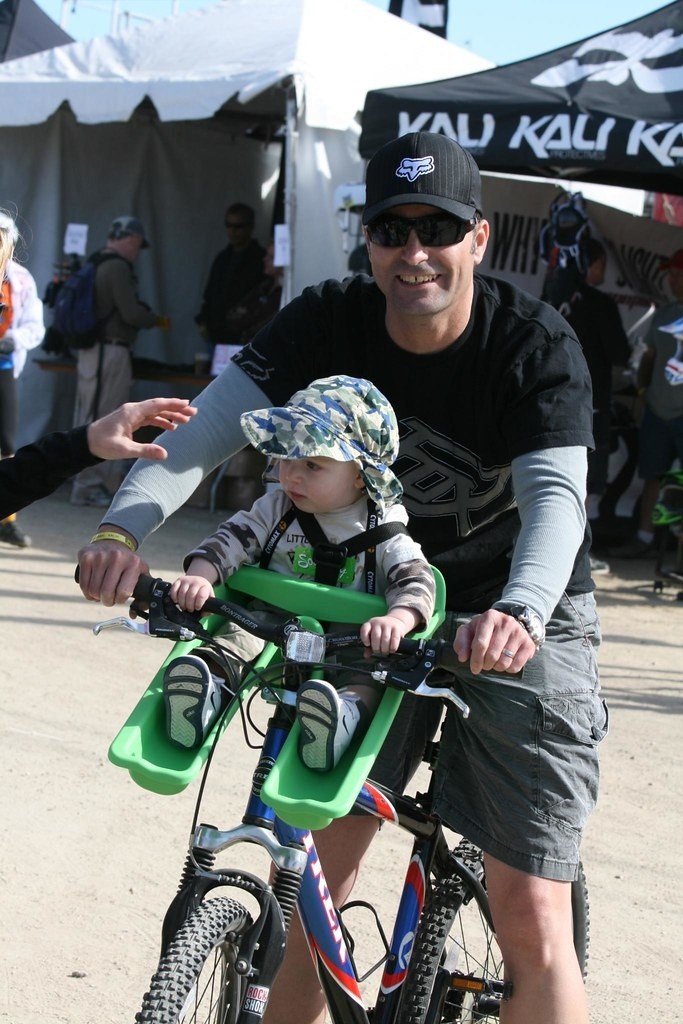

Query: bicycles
[{"left": 70, "top": 556, "right": 591, "bottom": 1024}]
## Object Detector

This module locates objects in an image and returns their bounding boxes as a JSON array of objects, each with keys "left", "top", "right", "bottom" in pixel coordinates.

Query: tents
[
  {"left": 0, "top": 0, "right": 498, "bottom": 513},
  {"left": 358, "top": 0, "right": 683, "bottom": 531}
]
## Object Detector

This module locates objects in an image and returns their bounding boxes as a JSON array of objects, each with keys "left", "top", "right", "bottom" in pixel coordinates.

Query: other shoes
[
  {"left": 296, "top": 679, "right": 359, "bottom": 772},
  {"left": 162, "top": 655, "right": 222, "bottom": 749},
  {"left": 70, "top": 486, "right": 112, "bottom": 507},
  {"left": 0, "top": 521, "right": 31, "bottom": 547},
  {"left": 608, "top": 535, "right": 659, "bottom": 558}
]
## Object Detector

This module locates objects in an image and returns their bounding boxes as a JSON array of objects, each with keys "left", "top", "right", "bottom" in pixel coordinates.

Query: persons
[
  {"left": 537, "top": 240, "right": 683, "bottom": 555},
  {"left": 69, "top": 215, "right": 173, "bottom": 508},
  {"left": 80, "top": 131, "right": 611, "bottom": 1024},
  {"left": 0, "top": 397, "right": 198, "bottom": 522},
  {"left": 0, "top": 213, "right": 47, "bottom": 549},
  {"left": 162, "top": 374, "right": 438, "bottom": 773},
  {"left": 197, "top": 201, "right": 275, "bottom": 386}
]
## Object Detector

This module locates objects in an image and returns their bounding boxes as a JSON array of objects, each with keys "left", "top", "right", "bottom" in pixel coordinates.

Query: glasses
[
  {"left": 225, "top": 222, "right": 248, "bottom": 228},
  {"left": 366, "top": 213, "right": 477, "bottom": 248}
]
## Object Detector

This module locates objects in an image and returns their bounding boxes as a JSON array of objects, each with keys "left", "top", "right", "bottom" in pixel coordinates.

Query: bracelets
[{"left": 90, "top": 532, "right": 135, "bottom": 552}]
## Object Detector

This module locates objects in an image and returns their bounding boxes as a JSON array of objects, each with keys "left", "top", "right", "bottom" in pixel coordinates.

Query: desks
[{"left": 133, "top": 381, "right": 231, "bottom": 512}]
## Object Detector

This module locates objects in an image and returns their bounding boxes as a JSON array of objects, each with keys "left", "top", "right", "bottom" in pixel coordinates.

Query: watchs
[{"left": 490, "top": 602, "right": 545, "bottom": 651}]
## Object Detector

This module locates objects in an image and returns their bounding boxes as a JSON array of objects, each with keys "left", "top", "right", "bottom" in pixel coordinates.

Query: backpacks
[{"left": 53, "top": 252, "right": 133, "bottom": 338}]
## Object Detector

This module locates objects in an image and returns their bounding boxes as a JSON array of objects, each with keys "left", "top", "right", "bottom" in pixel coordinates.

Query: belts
[{"left": 96, "top": 340, "right": 129, "bottom": 347}]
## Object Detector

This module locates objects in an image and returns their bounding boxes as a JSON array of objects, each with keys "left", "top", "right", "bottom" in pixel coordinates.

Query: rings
[{"left": 502, "top": 649, "right": 515, "bottom": 658}]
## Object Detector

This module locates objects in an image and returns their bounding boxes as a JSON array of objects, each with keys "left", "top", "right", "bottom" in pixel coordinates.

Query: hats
[
  {"left": 363, "top": 131, "right": 483, "bottom": 224},
  {"left": 109, "top": 216, "right": 149, "bottom": 250},
  {"left": 239, "top": 375, "right": 404, "bottom": 507}
]
